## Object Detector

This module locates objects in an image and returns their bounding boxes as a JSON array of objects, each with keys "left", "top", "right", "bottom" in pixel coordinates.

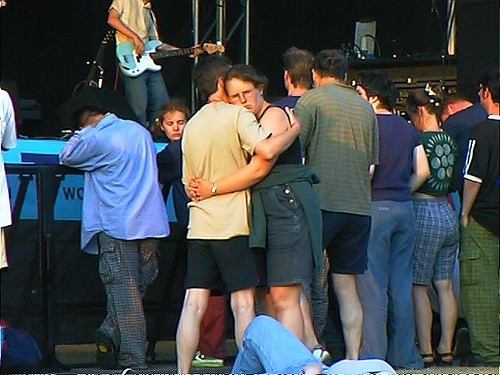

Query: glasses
[
  {"left": 476, "top": 86, "right": 488, "bottom": 92},
  {"left": 228, "top": 87, "right": 258, "bottom": 103}
]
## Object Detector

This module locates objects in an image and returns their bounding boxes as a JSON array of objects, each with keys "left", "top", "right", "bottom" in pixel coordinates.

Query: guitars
[
  {"left": 116, "top": 40, "right": 225, "bottom": 78},
  {"left": 72, "top": 29, "right": 115, "bottom": 96}
]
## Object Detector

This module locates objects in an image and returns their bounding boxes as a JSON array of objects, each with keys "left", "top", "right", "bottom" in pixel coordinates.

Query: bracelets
[{"left": 291, "top": 121, "right": 303, "bottom": 130}]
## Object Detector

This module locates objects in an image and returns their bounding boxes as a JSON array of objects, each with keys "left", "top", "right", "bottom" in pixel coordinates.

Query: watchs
[{"left": 211, "top": 182, "right": 217, "bottom": 196}]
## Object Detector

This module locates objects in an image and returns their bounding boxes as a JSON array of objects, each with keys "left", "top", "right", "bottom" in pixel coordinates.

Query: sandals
[{"left": 420, "top": 348, "right": 454, "bottom": 369}]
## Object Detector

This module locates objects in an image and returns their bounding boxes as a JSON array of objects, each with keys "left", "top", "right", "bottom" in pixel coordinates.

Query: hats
[{"left": 60, "top": 87, "right": 126, "bottom": 128}]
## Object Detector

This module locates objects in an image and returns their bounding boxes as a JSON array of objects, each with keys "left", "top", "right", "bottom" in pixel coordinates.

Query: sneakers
[
  {"left": 460, "top": 352, "right": 500, "bottom": 368},
  {"left": 95, "top": 329, "right": 117, "bottom": 369},
  {"left": 311, "top": 345, "right": 332, "bottom": 366},
  {"left": 121, "top": 368, "right": 136, "bottom": 375},
  {"left": 452, "top": 328, "right": 471, "bottom": 361},
  {"left": 190, "top": 351, "right": 224, "bottom": 367},
  {"left": 115, "top": 362, "right": 148, "bottom": 369}
]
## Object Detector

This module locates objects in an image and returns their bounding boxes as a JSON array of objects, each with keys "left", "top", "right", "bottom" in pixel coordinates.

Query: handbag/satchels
[{"left": 1, "top": 318, "right": 42, "bottom": 373}]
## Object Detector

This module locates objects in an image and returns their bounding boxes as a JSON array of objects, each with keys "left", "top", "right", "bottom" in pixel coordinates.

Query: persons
[
  {"left": 356, "top": 73, "right": 430, "bottom": 370},
  {"left": 294, "top": 49, "right": 381, "bottom": 360},
  {"left": 184, "top": 63, "right": 332, "bottom": 366},
  {"left": 459, "top": 67, "right": 500, "bottom": 367},
  {"left": 231, "top": 315, "right": 397, "bottom": 374},
  {"left": 437, "top": 95, "right": 489, "bottom": 203},
  {"left": 428, "top": 190, "right": 471, "bottom": 358},
  {"left": 59, "top": 105, "right": 171, "bottom": 370},
  {"left": 272, "top": 46, "right": 315, "bottom": 109},
  {"left": 0, "top": 87, "right": 16, "bottom": 322},
  {"left": 177, "top": 54, "right": 303, "bottom": 375},
  {"left": 107, "top": 0, "right": 200, "bottom": 126},
  {"left": 406, "top": 90, "right": 460, "bottom": 366},
  {"left": 142, "top": 100, "right": 227, "bottom": 368}
]
